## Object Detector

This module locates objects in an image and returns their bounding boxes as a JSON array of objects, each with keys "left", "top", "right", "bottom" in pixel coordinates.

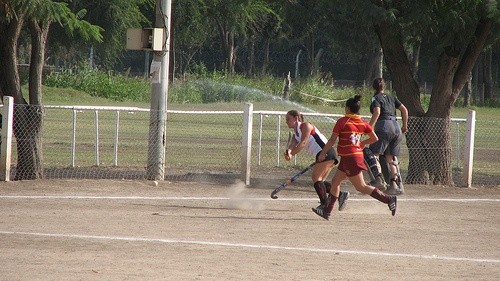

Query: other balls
[{"left": 153, "top": 181, "right": 159, "bottom": 186}]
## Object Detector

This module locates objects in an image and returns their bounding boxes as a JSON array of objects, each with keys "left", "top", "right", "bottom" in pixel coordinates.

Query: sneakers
[
  {"left": 368, "top": 178, "right": 386, "bottom": 190},
  {"left": 312, "top": 208, "right": 331, "bottom": 221},
  {"left": 339, "top": 192, "right": 350, "bottom": 211},
  {"left": 388, "top": 196, "right": 397, "bottom": 216},
  {"left": 385, "top": 188, "right": 403, "bottom": 195}
]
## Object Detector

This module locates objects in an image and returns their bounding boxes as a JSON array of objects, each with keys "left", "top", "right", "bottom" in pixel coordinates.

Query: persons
[
  {"left": 311, "top": 94, "right": 397, "bottom": 221},
  {"left": 362, "top": 78, "right": 408, "bottom": 195},
  {"left": 283, "top": 110, "right": 350, "bottom": 211}
]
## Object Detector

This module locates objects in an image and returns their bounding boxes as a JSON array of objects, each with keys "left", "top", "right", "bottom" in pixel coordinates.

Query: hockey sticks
[
  {"left": 271, "top": 161, "right": 316, "bottom": 200},
  {"left": 284, "top": 130, "right": 292, "bottom": 155}
]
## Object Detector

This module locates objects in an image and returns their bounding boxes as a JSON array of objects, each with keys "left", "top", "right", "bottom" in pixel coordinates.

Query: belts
[{"left": 386, "top": 118, "right": 395, "bottom": 120}]
